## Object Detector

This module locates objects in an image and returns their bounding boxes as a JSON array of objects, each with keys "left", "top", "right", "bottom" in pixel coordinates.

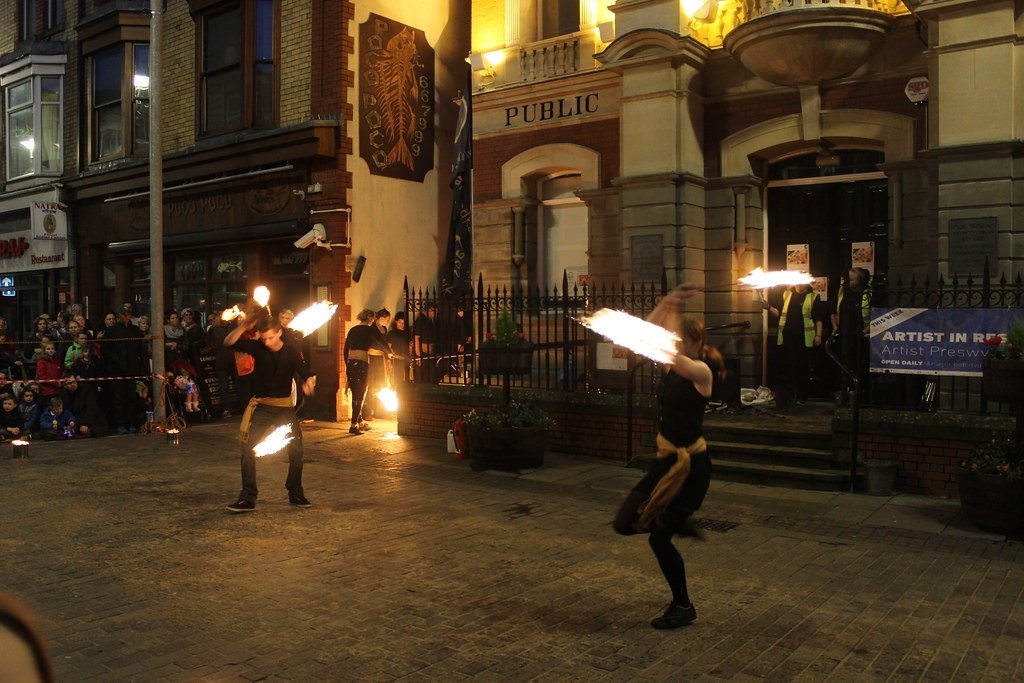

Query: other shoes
[
  {"left": 795, "top": 394, "right": 808, "bottom": 405},
  {"left": 130, "top": 426, "right": 137, "bottom": 433},
  {"left": 118, "top": 426, "right": 128, "bottom": 435},
  {"left": 221, "top": 410, "right": 232, "bottom": 420},
  {"left": 5, "top": 438, "right": 10, "bottom": 443},
  {"left": 0, "top": 435, "right": 5, "bottom": 441},
  {"left": 349, "top": 427, "right": 364, "bottom": 435}
]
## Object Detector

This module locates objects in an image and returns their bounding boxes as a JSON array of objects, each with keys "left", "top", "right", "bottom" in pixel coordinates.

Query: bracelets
[
  {"left": 767, "top": 304, "right": 771, "bottom": 310},
  {"left": 817, "top": 335, "right": 822, "bottom": 337}
]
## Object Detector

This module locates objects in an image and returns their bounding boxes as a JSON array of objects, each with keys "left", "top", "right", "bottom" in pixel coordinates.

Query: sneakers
[
  {"left": 225, "top": 498, "right": 255, "bottom": 513},
  {"left": 288, "top": 492, "right": 313, "bottom": 507},
  {"left": 651, "top": 601, "right": 697, "bottom": 629},
  {"left": 677, "top": 524, "right": 698, "bottom": 539}
]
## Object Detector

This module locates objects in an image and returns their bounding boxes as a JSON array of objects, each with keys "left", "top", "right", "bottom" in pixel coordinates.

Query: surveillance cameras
[{"left": 294, "top": 229, "right": 321, "bottom": 249}]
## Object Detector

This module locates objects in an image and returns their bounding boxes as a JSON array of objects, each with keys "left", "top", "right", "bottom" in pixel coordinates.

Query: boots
[
  {"left": 192, "top": 401, "right": 201, "bottom": 412},
  {"left": 184, "top": 402, "right": 194, "bottom": 412}
]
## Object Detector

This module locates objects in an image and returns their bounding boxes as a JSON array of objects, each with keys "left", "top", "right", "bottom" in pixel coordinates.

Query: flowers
[
  {"left": 959, "top": 434, "right": 1024, "bottom": 479},
  {"left": 484, "top": 307, "right": 526, "bottom": 345},
  {"left": 983, "top": 321, "right": 1024, "bottom": 360},
  {"left": 464, "top": 400, "right": 557, "bottom": 431}
]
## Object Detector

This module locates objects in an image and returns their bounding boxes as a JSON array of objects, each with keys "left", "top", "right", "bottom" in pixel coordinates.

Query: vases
[
  {"left": 479, "top": 341, "right": 533, "bottom": 374},
  {"left": 954, "top": 460, "right": 1024, "bottom": 533},
  {"left": 982, "top": 358, "right": 1024, "bottom": 404},
  {"left": 466, "top": 422, "right": 547, "bottom": 470}
]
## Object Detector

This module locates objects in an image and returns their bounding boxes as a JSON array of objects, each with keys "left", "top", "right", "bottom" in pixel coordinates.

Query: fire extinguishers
[{"left": 453, "top": 408, "right": 471, "bottom": 459}]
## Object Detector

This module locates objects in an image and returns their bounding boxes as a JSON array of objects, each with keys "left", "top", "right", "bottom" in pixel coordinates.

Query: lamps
[
  {"left": 598, "top": 21, "right": 614, "bottom": 42},
  {"left": 470, "top": 52, "right": 494, "bottom": 76},
  {"left": 512, "top": 207, "right": 526, "bottom": 267},
  {"left": 734, "top": 187, "right": 750, "bottom": 260},
  {"left": 889, "top": 174, "right": 906, "bottom": 247},
  {"left": 688, "top": 0, "right": 718, "bottom": 31}
]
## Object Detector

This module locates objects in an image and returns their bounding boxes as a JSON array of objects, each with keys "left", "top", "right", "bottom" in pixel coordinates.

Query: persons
[
  {"left": 830, "top": 267, "right": 872, "bottom": 391},
  {"left": 0, "top": 304, "right": 220, "bottom": 442},
  {"left": 760, "top": 284, "right": 823, "bottom": 405},
  {"left": 342, "top": 304, "right": 438, "bottom": 435},
  {"left": 277, "top": 308, "right": 308, "bottom": 417},
  {"left": 612, "top": 281, "right": 727, "bottom": 629},
  {"left": 223, "top": 313, "right": 316, "bottom": 510}
]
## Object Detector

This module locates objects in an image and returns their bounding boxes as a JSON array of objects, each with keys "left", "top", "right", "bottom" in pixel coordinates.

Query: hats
[
  {"left": 181, "top": 307, "right": 195, "bottom": 318},
  {"left": 118, "top": 305, "right": 132, "bottom": 313}
]
